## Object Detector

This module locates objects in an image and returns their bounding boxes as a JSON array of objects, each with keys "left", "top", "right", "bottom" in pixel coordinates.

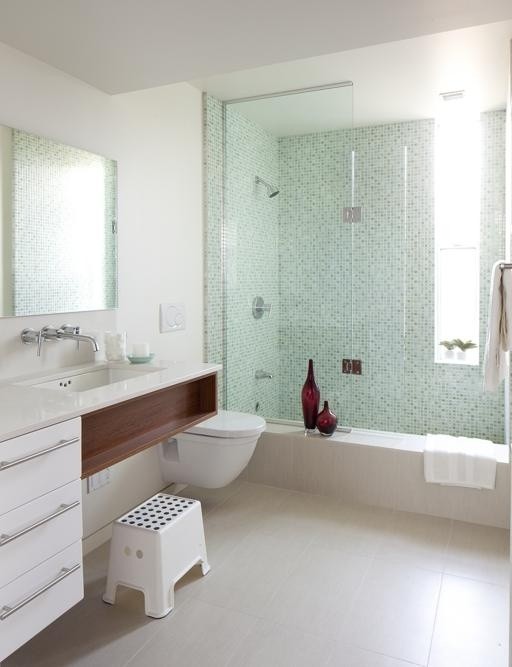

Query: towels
[
  {"left": 481, "top": 257, "right": 511, "bottom": 395},
  {"left": 422, "top": 432, "right": 498, "bottom": 491}
]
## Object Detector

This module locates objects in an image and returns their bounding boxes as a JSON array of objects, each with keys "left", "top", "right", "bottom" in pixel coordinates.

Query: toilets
[{"left": 156, "top": 410, "right": 266, "bottom": 489}]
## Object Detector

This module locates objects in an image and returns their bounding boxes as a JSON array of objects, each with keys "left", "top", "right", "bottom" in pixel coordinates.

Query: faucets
[
  {"left": 256, "top": 369, "right": 272, "bottom": 380},
  {"left": 42, "top": 326, "right": 98, "bottom": 353}
]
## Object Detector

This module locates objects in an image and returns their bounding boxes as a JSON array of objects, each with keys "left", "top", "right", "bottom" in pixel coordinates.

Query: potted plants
[{"left": 437, "top": 336, "right": 478, "bottom": 363}]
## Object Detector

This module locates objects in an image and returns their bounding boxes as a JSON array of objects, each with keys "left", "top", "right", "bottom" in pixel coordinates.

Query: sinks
[{"left": 11, "top": 364, "right": 166, "bottom": 394}]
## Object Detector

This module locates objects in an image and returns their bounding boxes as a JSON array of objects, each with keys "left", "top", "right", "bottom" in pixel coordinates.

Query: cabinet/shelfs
[{"left": 0, "top": 414, "right": 85, "bottom": 662}]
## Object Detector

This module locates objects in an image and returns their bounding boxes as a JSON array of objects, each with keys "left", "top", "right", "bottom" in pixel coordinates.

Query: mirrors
[{"left": 11, "top": 128, "right": 122, "bottom": 319}]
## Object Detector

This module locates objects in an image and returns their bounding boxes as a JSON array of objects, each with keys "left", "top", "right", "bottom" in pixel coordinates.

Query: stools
[{"left": 101, "top": 490, "right": 212, "bottom": 620}]
